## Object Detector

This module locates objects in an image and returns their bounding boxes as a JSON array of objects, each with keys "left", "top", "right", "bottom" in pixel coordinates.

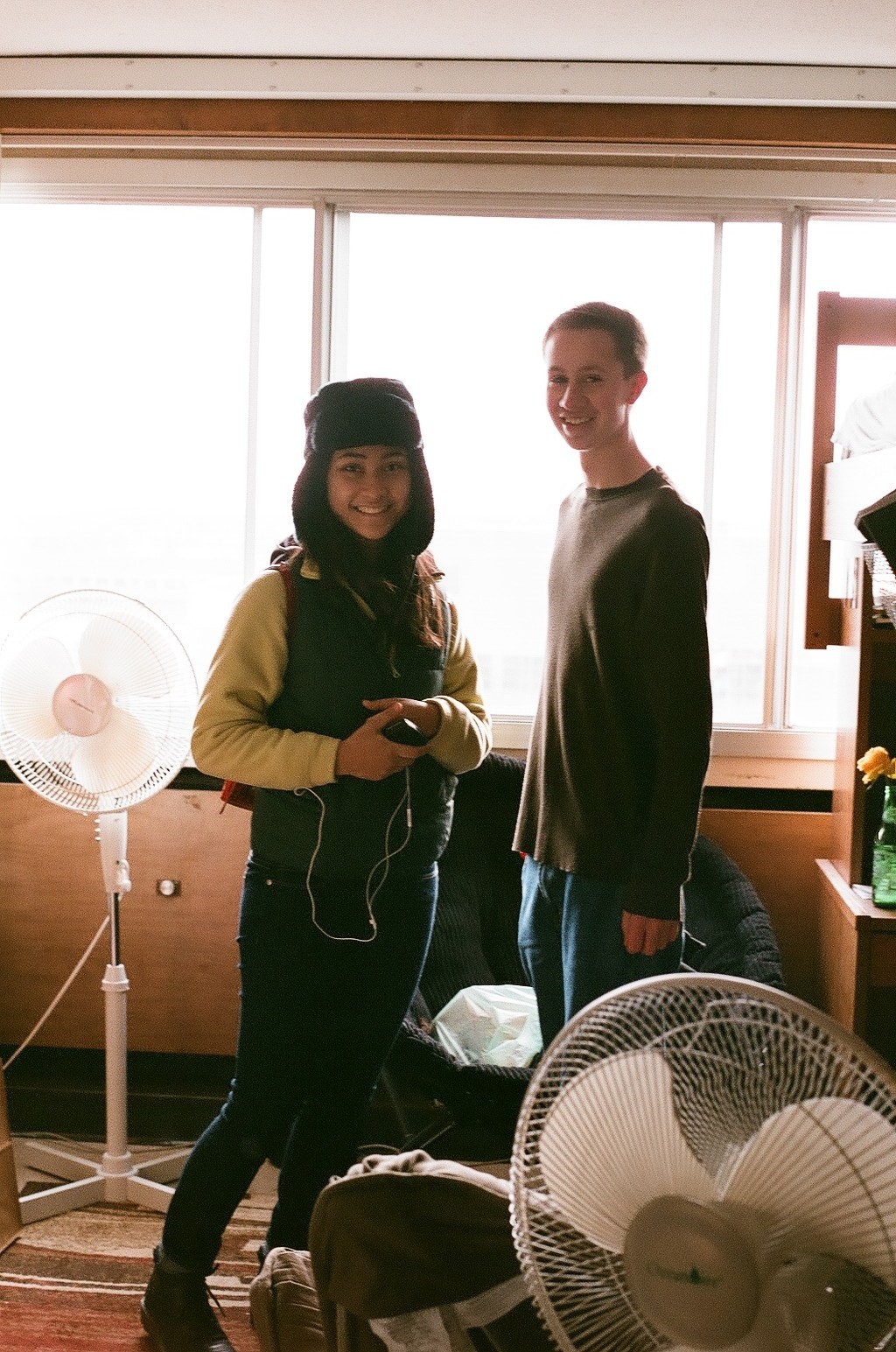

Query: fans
[
  {"left": 0, "top": 582, "right": 201, "bottom": 1224},
  {"left": 510, "top": 972, "right": 896, "bottom": 1352}
]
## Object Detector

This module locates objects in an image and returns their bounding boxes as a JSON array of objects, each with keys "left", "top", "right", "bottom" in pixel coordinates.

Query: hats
[{"left": 292, "top": 378, "right": 435, "bottom": 559}]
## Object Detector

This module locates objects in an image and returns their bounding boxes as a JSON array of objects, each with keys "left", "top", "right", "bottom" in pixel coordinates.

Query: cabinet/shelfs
[{"left": 815, "top": 490, "right": 896, "bottom": 1100}]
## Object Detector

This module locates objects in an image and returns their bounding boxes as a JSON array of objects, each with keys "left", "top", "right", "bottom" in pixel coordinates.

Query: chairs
[{"left": 371, "top": 750, "right": 787, "bottom": 1170}]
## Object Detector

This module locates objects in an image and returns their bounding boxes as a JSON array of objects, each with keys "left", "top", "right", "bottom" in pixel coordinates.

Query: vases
[{"left": 872, "top": 788, "right": 896, "bottom": 908}]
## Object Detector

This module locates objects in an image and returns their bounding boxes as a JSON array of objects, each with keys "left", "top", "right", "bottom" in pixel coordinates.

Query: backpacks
[
  {"left": 247, "top": 1247, "right": 327, "bottom": 1352},
  {"left": 307, "top": 1149, "right": 596, "bottom": 1352}
]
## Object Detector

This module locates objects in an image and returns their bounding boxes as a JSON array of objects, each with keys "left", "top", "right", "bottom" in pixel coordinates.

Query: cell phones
[{"left": 379, "top": 716, "right": 428, "bottom": 747}]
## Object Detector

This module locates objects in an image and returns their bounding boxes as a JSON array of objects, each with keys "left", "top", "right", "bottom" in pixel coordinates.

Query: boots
[{"left": 140, "top": 1246, "right": 236, "bottom": 1352}]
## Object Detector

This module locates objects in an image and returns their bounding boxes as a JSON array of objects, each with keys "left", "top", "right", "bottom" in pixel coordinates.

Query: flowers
[{"left": 856, "top": 745, "right": 896, "bottom": 790}]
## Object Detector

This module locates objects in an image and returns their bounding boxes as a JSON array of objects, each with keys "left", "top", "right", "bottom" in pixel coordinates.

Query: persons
[
  {"left": 513, "top": 303, "right": 711, "bottom": 1060},
  {"left": 134, "top": 380, "right": 492, "bottom": 1352}
]
